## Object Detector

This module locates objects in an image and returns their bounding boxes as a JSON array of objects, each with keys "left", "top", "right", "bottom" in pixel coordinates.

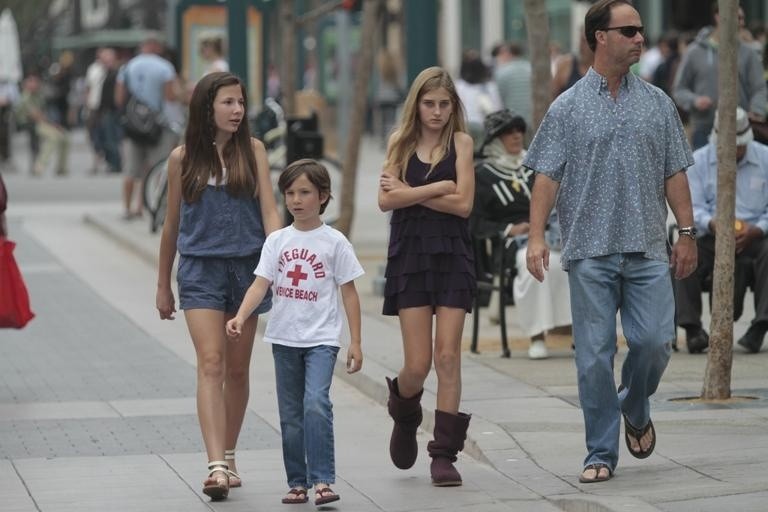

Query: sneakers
[
  {"left": 686, "top": 328, "right": 709, "bottom": 355},
  {"left": 738, "top": 323, "right": 766, "bottom": 355}
]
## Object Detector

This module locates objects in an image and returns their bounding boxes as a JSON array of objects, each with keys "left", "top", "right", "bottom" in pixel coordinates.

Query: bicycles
[{"left": 137, "top": 97, "right": 348, "bottom": 247}]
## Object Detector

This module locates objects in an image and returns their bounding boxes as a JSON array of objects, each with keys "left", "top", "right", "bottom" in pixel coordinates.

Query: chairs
[
  {"left": 670, "top": 272, "right": 713, "bottom": 351},
  {"left": 470, "top": 150, "right": 517, "bottom": 357}
]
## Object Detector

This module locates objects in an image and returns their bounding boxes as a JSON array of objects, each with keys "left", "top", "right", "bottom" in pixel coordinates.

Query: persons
[
  {"left": 455, "top": 1, "right": 766, "bottom": 363},
  {"left": 372, "top": 64, "right": 474, "bottom": 492},
  {"left": 155, "top": 70, "right": 284, "bottom": 503},
  {"left": 520, "top": 0, "right": 701, "bottom": 487},
  {"left": 225, "top": 156, "right": 367, "bottom": 507},
  {"left": 1, "top": 33, "right": 228, "bottom": 225}
]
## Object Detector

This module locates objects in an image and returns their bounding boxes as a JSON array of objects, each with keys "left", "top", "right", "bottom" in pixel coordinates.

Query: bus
[{"left": 43, "top": 30, "right": 172, "bottom": 129}]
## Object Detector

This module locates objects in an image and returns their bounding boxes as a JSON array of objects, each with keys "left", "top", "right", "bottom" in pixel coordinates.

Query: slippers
[
  {"left": 282, "top": 488, "right": 309, "bottom": 504},
  {"left": 617, "top": 383, "right": 656, "bottom": 460},
  {"left": 314, "top": 488, "right": 340, "bottom": 506},
  {"left": 579, "top": 463, "right": 616, "bottom": 484}
]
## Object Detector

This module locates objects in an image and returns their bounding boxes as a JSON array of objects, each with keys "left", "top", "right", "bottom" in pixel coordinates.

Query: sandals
[
  {"left": 203, "top": 460, "right": 230, "bottom": 502},
  {"left": 225, "top": 450, "right": 242, "bottom": 488}
]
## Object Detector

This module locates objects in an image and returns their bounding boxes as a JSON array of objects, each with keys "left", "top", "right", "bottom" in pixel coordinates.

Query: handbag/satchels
[
  {"left": 1, "top": 230, "right": 36, "bottom": 330},
  {"left": 119, "top": 62, "right": 162, "bottom": 148}
]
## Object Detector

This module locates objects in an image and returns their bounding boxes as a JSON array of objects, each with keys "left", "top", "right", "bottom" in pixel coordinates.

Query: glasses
[{"left": 600, "top": 23, "right": 645, "bottom": 40}]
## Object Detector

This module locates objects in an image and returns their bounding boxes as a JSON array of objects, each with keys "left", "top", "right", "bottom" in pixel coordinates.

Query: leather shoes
[{"left": 529, "top": 334, "right": 549, "bottom": 360}]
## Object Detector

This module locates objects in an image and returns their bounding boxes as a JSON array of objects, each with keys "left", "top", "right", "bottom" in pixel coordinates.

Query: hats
[
  {"left": 709, "top": 105, "right": 756, "bottom": 150},
  {"left": 480, "top": 107, "right": 527, "bottom": 161}
]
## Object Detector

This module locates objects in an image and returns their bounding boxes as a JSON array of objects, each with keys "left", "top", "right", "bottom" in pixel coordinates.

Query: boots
[
  {"left": 384, "top": 377, "right": 426, "bottom": 471},
  {"left": 427, "top": 404, "right": 472, "bottom": 487}
]
